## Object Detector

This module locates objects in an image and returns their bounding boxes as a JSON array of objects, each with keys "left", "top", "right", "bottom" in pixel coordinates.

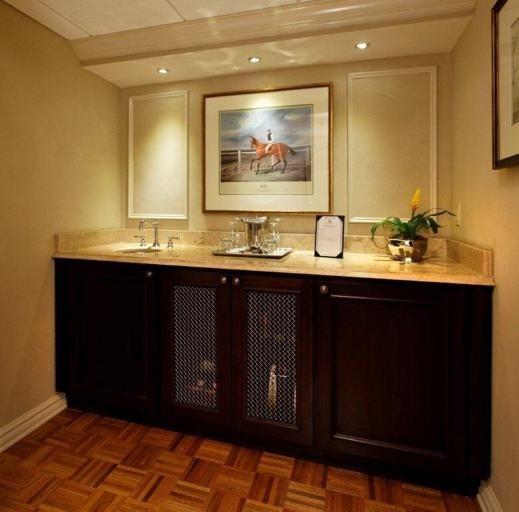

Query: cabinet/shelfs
[{"left": 52, "top": 251, "right": 495, "bottom": 503}]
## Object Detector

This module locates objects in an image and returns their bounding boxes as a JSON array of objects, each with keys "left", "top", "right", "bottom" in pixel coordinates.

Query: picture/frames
[
  {"left": 201, "top": 82, "right": 337, "bottom": 214},
  {"left": 489, "top": 1, "right": 518, "bottom": 170}
]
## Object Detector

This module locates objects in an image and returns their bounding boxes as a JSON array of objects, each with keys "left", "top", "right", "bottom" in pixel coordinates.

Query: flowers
[{"left": 370, "top": 189, "right": 453, "bottom": 240}]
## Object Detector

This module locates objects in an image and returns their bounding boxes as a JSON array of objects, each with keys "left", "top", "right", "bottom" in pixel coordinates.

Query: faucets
[{"left": 138, "top": 216, "right": 162, "bottom": 247}]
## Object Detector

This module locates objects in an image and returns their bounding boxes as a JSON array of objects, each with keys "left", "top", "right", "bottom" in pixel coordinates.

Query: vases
[{"left": 385, "top": 236, "right": 429, "bottom": 263}]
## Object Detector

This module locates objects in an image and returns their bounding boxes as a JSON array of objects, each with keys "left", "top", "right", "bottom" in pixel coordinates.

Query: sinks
[{"left": 115, "top": 248, "right": 163, "bottom": 254}]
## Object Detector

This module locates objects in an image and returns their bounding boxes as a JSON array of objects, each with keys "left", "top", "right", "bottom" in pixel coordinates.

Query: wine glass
[{"left": 223, "top": 213, "right": 282, "bottom": 252}]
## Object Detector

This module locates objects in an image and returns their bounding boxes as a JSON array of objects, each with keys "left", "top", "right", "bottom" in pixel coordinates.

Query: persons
[{"left": 264, "top": 130, "right": 273, "bottom": 154}]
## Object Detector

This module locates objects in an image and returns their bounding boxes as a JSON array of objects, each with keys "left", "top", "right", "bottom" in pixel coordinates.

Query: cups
[
  {"left": 262, "top": 240, "right": 278, "bottom": 255},
  {"left": 221, "top": 238, "right": 233, "bottom": 254}
]
